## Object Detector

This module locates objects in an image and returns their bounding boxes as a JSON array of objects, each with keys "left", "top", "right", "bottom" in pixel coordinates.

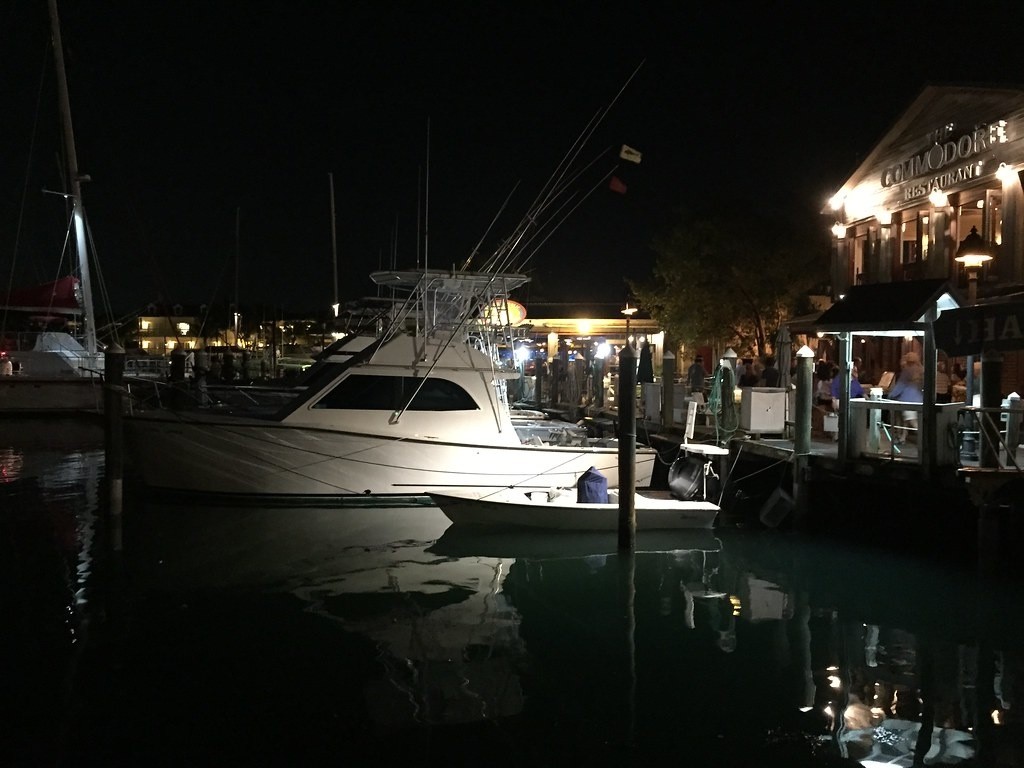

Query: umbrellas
[
  {"left": 636, "top": 339, "right": 654, "bottom": 384},
  {"left": 774, "top": 327, "right": 791, "bottom": 388},
  {"left": 557, "top": 337, "right": 569, "bottom": 371}
]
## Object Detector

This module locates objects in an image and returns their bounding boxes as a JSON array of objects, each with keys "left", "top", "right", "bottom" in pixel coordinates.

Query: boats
[
  {"left": 101, "top": 55, "right": 657, "bottom": 497},
  {"left": 424, "top": 524, "right": 726, "bottom": 559},
  {"left": 1, "top": 273, "right": 168, "bottom": 417},
  {"left": 423, "top": 440, "right": 725, "bottom": 526}
]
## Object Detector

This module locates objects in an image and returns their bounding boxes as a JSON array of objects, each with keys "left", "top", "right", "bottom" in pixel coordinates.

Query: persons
[
  {"left": 0, "top": 354, "right": 12, "bottom": 376},
  {"left": 851, "top": 356, "right": 868, "bottom": 383},
  {"left": 950, "top": 363, "right": 964, "bottom": 384},
  {"left": 829, "top": 364, "right": 870, "bottom": 443},
  {"left": 790, "top": 357, "right": 839, "bottom": 382},
  {"left": 936, "top": 361, "right": 951, "bottom": 403},
  {"left": 684, "top": 356, "right": 715, "bottom": 402},
  {"left": 886, "top": 351, "right": 924, "bottom": 445},
  {"left": 735, "top": 356, "right": 780, "bottom": 388}
]
